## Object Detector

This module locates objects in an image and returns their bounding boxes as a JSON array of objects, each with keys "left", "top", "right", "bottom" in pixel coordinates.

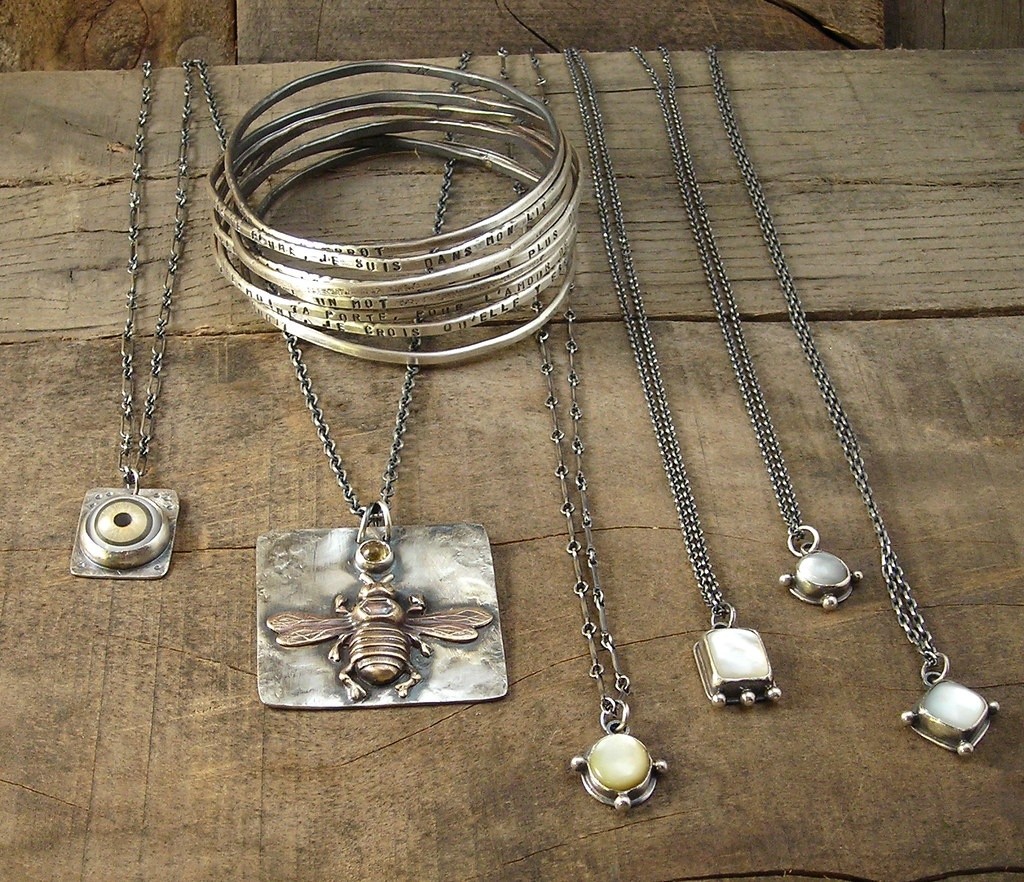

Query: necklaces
[
  {"left": 697, "top": 46, "right": 1000, "bottom": 756},
  {"left": 627, "top": 44, "right": 865, "bottom": 610},
  {"left": 559, "top": 47, "right": 784, "bottom": 710},
  {"left": 69, "top": 59, "right": 191, "bottom": 579},
  {"left": 189, "top": 51, "right": 509, "bottom": 709},
  {"left": 498, "top": 44, "right": 669, "bottom": 813},
  {"left": 202, "top": 61, "right": 583, "bottom": 366}
]
